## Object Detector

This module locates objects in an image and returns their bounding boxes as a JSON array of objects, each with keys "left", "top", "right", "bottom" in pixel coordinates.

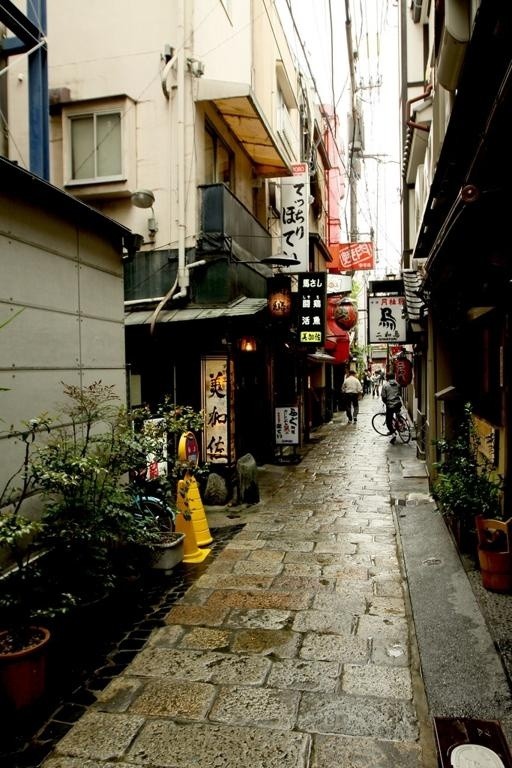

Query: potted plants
[
  {"left": 27, "top": 379, "right": 206, "bottom": 575},
  {"left": 0, "top": 413, "right": 81, "bottom": 709},
  {"left": 0, "top": 379, "right": 207, "bottom": 709}
]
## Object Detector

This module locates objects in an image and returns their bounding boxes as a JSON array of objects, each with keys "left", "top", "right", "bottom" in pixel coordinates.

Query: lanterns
[
  {"left": 393, "top": 356, "right": 414, "bottom": 387},
  {"left": 268, "top": 291, "right": 292, "bottom": 316},
  {"left": 334, "top": 298, "right": 358, "bottom": 332}
]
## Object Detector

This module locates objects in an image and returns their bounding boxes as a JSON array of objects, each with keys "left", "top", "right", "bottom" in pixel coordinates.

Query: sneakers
[
  {"left": 347, "top": 417, "right": 358, "bottom": 424},
  {"left": 389, "top": 436, "right": 396, "bottom": 443}
]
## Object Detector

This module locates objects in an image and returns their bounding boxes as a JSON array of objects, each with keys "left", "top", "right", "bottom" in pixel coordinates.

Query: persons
[
  {"left": 357, "top": 367, "right": 386, "bottom": 400},
  {"left": 339, "top": 369, "right": 365, "bottom": 425},
  {"left": 344, "top": 370, "right": 350, "bottom": 380},
  {"left": 380, "top": 372, "right": 404, "bottom": 444}
]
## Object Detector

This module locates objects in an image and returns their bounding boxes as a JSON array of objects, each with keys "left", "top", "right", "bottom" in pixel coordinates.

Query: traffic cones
[
  {"left": 183, "top": 470, "right": 213, "bottom": 546},
  {"left": 171, "top": 482, "right": 211, "bottom": 566}
]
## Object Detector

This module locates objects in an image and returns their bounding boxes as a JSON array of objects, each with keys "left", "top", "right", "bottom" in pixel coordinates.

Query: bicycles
[
  {"left": 372, "top": 404, "right": 411, "bottom": 444},
  {"left": 120, "top": 484, "right": 175, "bottom": 531}
]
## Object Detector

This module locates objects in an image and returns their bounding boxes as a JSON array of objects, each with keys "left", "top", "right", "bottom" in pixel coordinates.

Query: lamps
[{"left": 131, "top": 189, "right": 157, "bottom": 234}]
